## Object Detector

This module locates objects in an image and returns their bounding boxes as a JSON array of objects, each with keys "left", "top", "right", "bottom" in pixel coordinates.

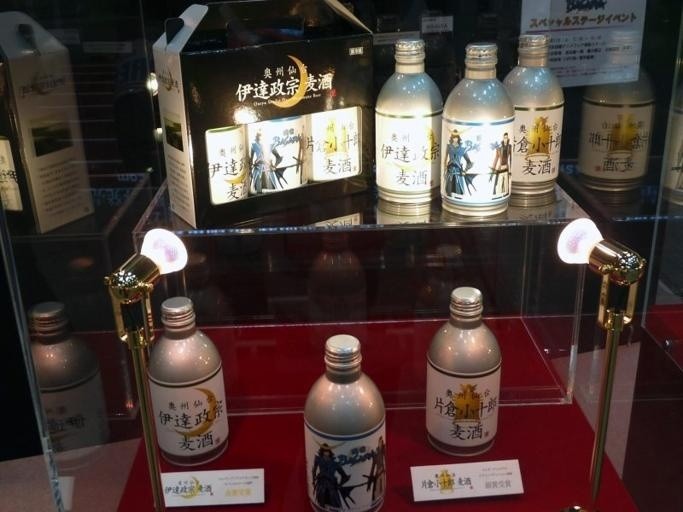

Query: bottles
[
  {"left": 144, "top": 299, "right": 227, "bottom": 467},
  {"left": 424, "top": 287, "right": 501, "bottom": 456},
  {"left": 664, "top": 83, "right": 682, "bottom": 215},
  {"left": 573, "top": 64, "right": 654, "bottom": 198},
  {"left": 303, "top": 332, "right": 388, "bottom": 512},
  {"left": 28, "top": 302, "right": 108, "bottom": 472},
  {"left": 205, "top": 108, "right": 360, "bottom": 209},
  {"left": 376, "top": 34, "right": 564, "bottom": 222}
]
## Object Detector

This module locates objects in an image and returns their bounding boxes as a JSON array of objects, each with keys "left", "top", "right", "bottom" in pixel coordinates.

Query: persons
[
  {"left": 312, "top": 442, "right": 351, "bottom": 512},
  {"left": 443, "top": 129, "right": 473, "bottom": 200},
  {"left": 249, "top": 133, "right": 282, "bottom": 194},
  {"left": 489, "top": 133, "right": 511, "bottom": 199},
  {"left": 367, "top": 436, "right": 386, "bottom": 500}
]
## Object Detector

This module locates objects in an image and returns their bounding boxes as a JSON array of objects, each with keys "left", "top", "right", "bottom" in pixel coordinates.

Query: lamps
[
  {"left": 556, "top": 217, "right": 647, "bottom": 501},
  {"left": 104, "top": 228, "right": 188, "bottom": 511}
]
